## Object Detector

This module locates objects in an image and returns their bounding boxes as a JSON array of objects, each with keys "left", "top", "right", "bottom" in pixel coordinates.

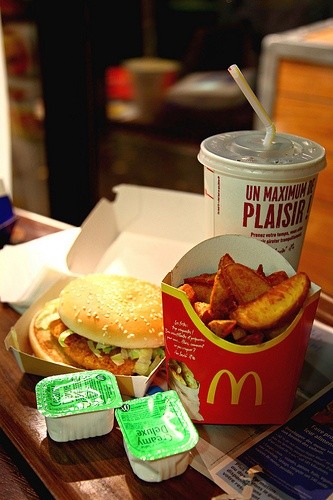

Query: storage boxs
[{"left": 165, "top": 70, "right": 248, "bottom": 147}]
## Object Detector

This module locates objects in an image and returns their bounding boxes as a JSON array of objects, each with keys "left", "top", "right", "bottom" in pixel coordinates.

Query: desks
[{"left": 0, "top": 207, "right": 333, "bottom": 500}]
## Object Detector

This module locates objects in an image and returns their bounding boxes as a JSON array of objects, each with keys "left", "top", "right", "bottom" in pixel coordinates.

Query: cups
[
  {"left": 126, "top": 58, "right": 178, "bottom": 123},
  {"left": 196, "top": 129, "right": 326, "bottom": 275}
]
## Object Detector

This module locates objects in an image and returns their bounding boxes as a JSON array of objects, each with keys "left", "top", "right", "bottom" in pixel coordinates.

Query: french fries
[{"left": 177, "top": 253, "right": 311, "bottom": 346}]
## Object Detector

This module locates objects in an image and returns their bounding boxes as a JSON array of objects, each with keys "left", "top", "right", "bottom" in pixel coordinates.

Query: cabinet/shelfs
[{"left": 256, "top": 18, "right": 333, "bottom": 326}]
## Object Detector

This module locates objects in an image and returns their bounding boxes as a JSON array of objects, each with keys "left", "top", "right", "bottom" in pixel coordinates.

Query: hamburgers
[{"left": 28, "top": 273, "right": 163, "bottom": 376}]
